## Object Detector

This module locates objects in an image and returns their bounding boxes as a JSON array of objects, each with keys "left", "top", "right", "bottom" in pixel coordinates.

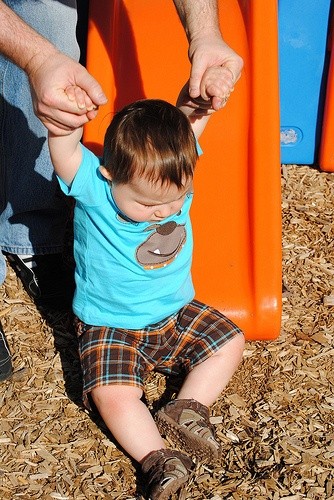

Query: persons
[
  {"left": 45, "top": 63, "right": 247, "bottom": 499},
  {"left": 0, "top": 1, "right": 246, "bottom": 379}
]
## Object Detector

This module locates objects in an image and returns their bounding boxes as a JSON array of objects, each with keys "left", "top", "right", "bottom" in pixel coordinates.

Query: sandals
[
  {"left": 154, "top": 399, "right": 221, "bottom": 465},
  {"left": 142, "top": 449, "right": 194, "bottom": 500}
]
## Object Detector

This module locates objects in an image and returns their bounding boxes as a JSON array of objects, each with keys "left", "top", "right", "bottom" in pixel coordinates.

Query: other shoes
[
  {"left": 0, "top": 321, "right": 12, "bottom": 381},
  {"left": 2, "top": 249, "right": 75, "bottom": 309}
]
